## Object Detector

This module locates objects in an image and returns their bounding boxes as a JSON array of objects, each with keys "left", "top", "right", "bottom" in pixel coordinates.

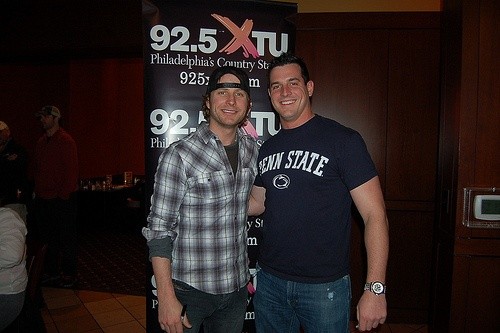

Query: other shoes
[
  {"left": 45, "top": 271, "right": 63, "bottom": 281},
  {"left": 63, "top": 275, "right": 79, "bottom": 287}
]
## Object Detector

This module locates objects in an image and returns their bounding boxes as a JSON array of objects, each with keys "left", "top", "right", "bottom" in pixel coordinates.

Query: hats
[
  {"left": 206, "top": 66, "right": 251, "bottom": 97},
  {"left": 35, "top": 105, "right": 61, "bottom": 118}
]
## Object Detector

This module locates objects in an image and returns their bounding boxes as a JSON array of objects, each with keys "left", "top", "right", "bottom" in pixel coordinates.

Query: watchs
[{"left": 364, "top": 281, "right": 386, "bottom": 296}]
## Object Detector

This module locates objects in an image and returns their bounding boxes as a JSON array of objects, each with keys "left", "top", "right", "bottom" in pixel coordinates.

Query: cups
[
  {"left": 80, "top": 176, "right": 113, "bottom": 191},
  {"left": 133, "top": 176, "right": 142, "bottom": 184}
]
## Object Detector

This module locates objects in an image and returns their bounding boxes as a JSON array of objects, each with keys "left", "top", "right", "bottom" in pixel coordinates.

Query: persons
[
  {"left": 0, "top": 152, "right": 29, "bottom": 331},
  {"left": 22, "top": 105, "right": 81, "bottom": 286},
  {"left": 141, "top": 66, "right": 258, "bottom": 333},
  {"left": 247, "top": 52, "right": 389, "bottom": 333},
  {"left": 0, "top": 120, "right": 25, "bottom": 206}
]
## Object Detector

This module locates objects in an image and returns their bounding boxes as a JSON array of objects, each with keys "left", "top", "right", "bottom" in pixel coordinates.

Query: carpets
[{"left": 39, "top": 229, "right": 148, "bottom": 296}]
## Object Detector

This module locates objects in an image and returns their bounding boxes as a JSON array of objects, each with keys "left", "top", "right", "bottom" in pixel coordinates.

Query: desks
[{"left": 78, "top": 188, "right": 131, "bottom": 231}]
